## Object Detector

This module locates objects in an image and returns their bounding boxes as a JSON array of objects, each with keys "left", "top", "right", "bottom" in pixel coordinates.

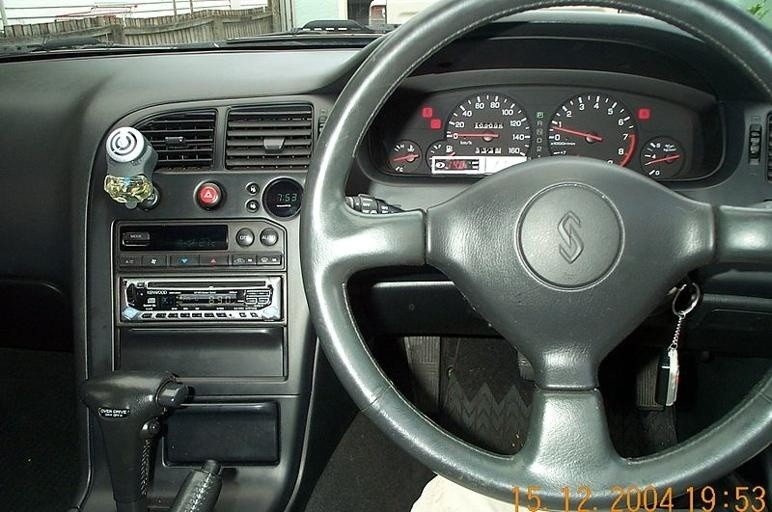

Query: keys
[{"left": 654, "top": 276, "right": 701, "bottom": 406}]
